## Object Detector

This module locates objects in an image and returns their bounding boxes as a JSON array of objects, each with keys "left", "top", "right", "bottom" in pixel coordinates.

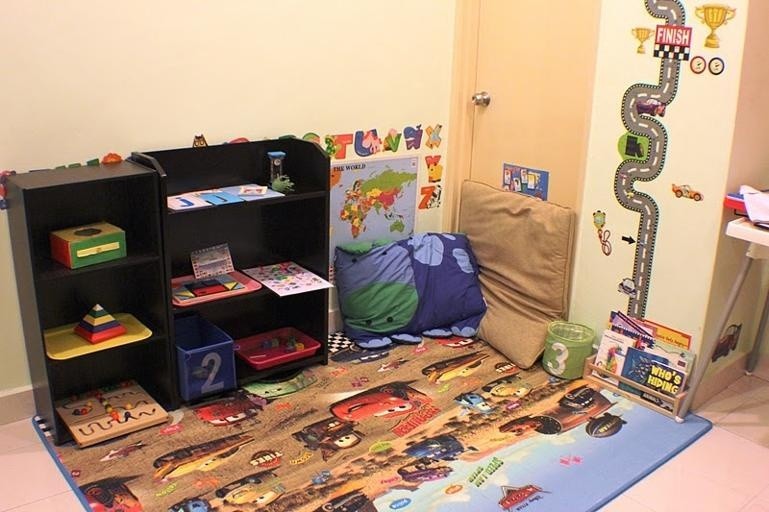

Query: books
[{"left": 595, "top": 310, "right": 697, "bottom": 414}]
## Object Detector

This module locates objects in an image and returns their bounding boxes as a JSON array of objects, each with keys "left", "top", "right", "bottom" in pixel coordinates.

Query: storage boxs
[
  {"left": 49, "top": 221, "right": 127, "bottom": 270},
  {"left": 174, "top": 309, "right": 238, "bottom": 402}
]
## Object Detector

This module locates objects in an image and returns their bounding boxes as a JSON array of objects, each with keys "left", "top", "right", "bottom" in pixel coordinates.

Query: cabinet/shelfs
[
  {"left": 5, "top": 159, "right": 179, "bottom": 446},
  {"left": 127, "top": 137, "right": 330, "bottom": 411}
]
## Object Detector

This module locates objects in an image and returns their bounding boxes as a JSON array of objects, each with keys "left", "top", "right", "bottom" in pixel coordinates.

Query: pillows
[
  {"left": 456, "top": 179, "right": 574, "bottom": 369},
  {"left": 335, "top": 230, "right": 490, "bottom": 352}
]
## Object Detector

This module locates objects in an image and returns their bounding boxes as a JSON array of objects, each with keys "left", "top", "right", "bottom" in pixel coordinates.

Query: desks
[{"left": 676, "top": 217, "right": 769, "bottom": 424}]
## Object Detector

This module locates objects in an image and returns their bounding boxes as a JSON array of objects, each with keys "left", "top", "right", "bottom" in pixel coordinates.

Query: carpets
[{"left": 32, "top": 330, "right": 715, "bottom": 512}]
